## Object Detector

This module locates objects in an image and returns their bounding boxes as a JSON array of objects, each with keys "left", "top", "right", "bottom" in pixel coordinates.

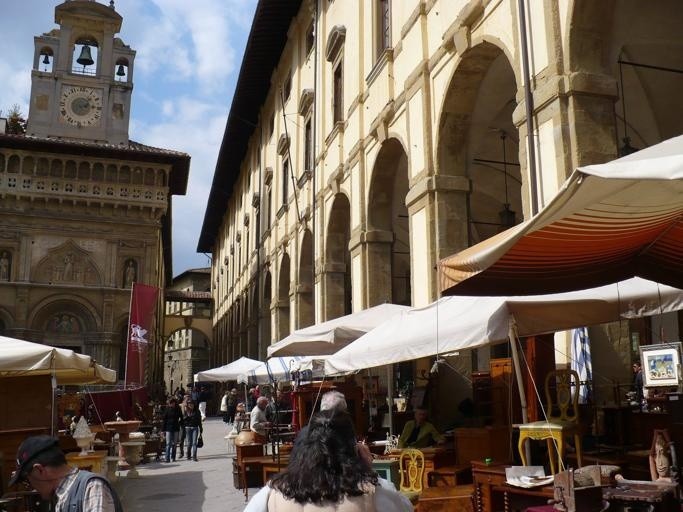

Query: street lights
[{"left": 197, "top": 435, "right": 203, "bottom": 448}]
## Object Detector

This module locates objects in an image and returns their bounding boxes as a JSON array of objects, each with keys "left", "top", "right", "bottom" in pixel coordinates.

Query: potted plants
[{"left": 638, "top": 341, "right": 683, "bottom": 388}]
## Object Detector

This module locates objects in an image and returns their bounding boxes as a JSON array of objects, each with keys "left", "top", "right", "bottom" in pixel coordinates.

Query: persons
[
  {"left": 243, "top": 405, "right": 415, "bottom": 511},
  {"left": 68, "top": 381, "right": 295, "bottom": 463},
  {"left": 63, "top": 250, "right": 75, "bottom": 283},
  {"left": 124, "top": 259, "right": 136, "bottom": 288},
  {"left": 397, "top": 406, "right": 446, "bottom": 449},
  {"left": 54, "top": 312, "right": 73, "bottom": 335},
  {"left": 7, "top": 433, "right": 123, "bottom": 511},
  {"left": 632, "top": 361, "right": 644, "bottom": 400},
  {"left": 49, "top": 316, "right": 61, "bottom": 335},
  {"left": 454, "top": 395, "right": 496, "bottom": 427},
  {"left": 71, "top": 316, "right": 80, "bottom": 334},
  {"left": 293, "top": 390, "right": 357, "bottom": 444},
  {"left": 0, "top": 250, "right": 9, "bottom": 282}
]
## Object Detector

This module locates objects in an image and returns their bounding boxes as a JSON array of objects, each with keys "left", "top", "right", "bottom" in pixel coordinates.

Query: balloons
[{"left": 59, "top": 85, "right": 104, "bottom": 130}]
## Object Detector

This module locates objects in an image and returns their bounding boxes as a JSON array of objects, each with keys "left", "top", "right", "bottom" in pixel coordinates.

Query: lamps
[
  {"left": 511, "top": 367, "right": 580, "bottom": 475},
  {"left": 383, "top": 425, "right": 496, "bottom": 512}
]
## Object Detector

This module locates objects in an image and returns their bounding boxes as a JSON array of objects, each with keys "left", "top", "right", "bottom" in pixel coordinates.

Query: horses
[{"left": 354, "top": 447, "right": 360, "bottom": 457}]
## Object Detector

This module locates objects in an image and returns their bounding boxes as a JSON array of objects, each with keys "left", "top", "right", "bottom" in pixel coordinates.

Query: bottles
[{"left": 8, "top": 436, "right": 59, "bottom": 488}]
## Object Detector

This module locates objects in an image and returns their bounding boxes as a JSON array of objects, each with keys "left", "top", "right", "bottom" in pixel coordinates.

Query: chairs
[
  {"left": 187, "top": 457, "right": 191, "bottom": 460},
  {"left": 164, "top": 460, "right": 170, "bottom": 463},
  {"left": 179, "top": 452, "right": 184, "bottom": 458},
  {"left": 193, "top": 457, "right": 198, "bottom": 460},
  {"left": 170, "top": 458, "right": 176, "bottom": 462}
]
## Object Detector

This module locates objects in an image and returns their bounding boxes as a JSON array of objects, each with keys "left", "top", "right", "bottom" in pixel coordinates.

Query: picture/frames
[{"left": 470, "top": 455, "right": 682, "bottom": 512}]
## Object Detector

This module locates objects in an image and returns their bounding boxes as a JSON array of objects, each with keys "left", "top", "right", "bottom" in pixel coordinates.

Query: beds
[
  {"left": 441, "top": 135, "right": 681, "bottom": 294},
  {"left": 237, "top": 351, "right": 336, "bottom": 387},
  {"left": 0, "top": 334, "right": 116, "bottom": 438},
  {"left": 322, "top": 273, "right": 682, "bottom": 466},
  {"left": 193, "top": 357, "right": 268, "bottom": 412},
  {"left": 265, "top": 303, "right": 421, "bottom": 437}
]
